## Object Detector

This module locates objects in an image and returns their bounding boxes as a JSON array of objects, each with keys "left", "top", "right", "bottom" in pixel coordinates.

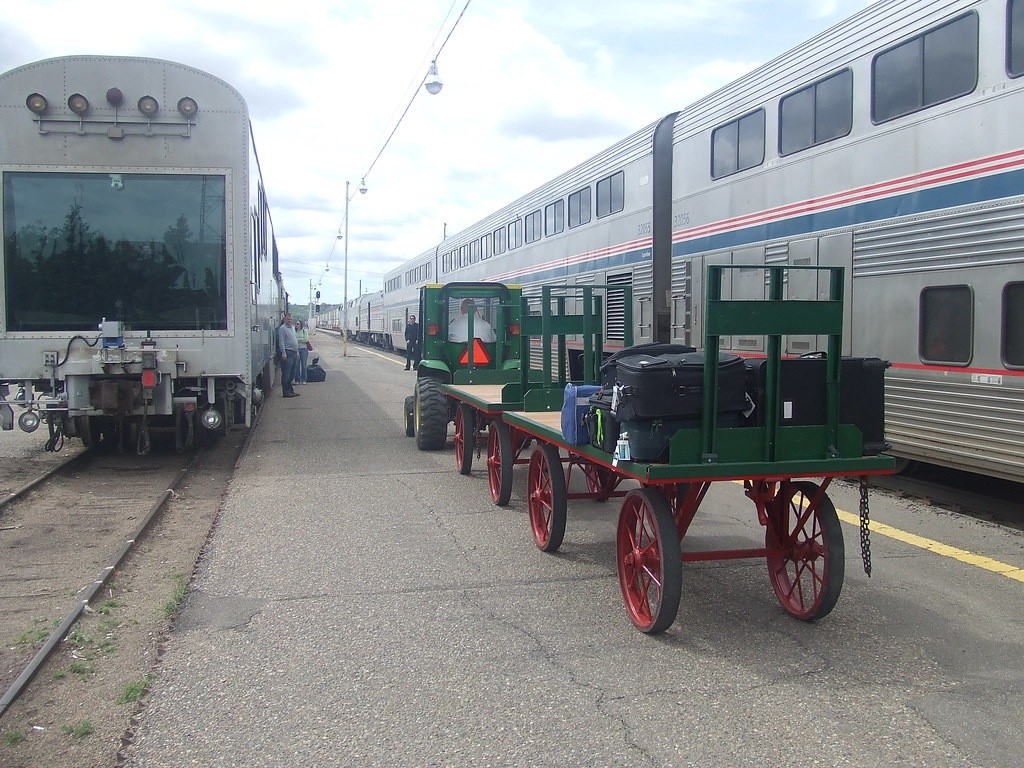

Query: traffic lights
[
  {"left": 317, "top": 291, "right": 320, "bottom": 298},
  {"left": 316, "top": 305, "right": 320, "bottom": 312}
]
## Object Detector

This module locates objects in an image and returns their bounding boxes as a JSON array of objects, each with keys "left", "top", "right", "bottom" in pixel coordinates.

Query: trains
[
  {"left": 317, "top": 0, "right": 1024, "bottom": 485},
  {"left": 0, "top": 55, "right": 288, "bottom": 454}
]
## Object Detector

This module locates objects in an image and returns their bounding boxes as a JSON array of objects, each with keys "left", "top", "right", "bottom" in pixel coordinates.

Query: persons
[
  {"left": 276, "top": 313, "right": 310, "bottom": 398},
  {"left": 449, "top": 298, "right": 498, "bottom": 343},
  {"left": 403, "top": 313, "right": 420, "bottom": 371}
]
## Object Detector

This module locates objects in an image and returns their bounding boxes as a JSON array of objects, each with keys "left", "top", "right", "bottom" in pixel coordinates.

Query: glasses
[{"left": 295, "top": 323, "right": 301, "bottom": 325}]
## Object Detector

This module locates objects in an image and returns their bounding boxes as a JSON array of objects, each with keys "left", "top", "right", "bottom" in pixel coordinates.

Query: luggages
[{"left": 600, "top": 341, "right": 893, "bottom": 467}]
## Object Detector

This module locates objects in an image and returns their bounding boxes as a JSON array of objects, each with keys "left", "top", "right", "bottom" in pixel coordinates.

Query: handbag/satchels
[
  {"left": 561, "top": 381, "right": 604, "bottom": 448},
  {"left": 583, "top": 390, "right": 619, "bottom": 453},
  {"left": 303, "top": 329, "right": 313, "bottom": 351}
]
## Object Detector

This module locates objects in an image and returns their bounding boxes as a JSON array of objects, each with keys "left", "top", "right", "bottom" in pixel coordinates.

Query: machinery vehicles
[{"left": 403, "top": 264, "right": 896, "bottom": 634}]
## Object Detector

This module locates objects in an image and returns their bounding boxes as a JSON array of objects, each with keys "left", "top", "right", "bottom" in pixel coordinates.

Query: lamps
[
  {"left": 425, "top": 62, "right": 443, "bottom": 95},
  {"left": 324, "top": 264, "right": 330, "bottom": 272},
  {"left": 365, "top": 288, "right": 367, "bottom": 292},
  {"left": 313, "top": 284, "right": 317, "bottom": 290},
  {"left": 358, "top": 178, "right": 368, "bottom": 194},
  {"left": 318, "top": 279, "right": 322, "bottom": 286},
  {"left": 336, "top": 228, "right": 343, "bottom": 240}
]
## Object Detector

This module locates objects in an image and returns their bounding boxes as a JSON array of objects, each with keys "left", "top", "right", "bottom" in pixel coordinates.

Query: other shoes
[
  {"left": 293, "top": 381, "right": 300, "bottom": 385},
  {"left": 283, "top": 393, "right": 296, "bottom": 397},
  {"left": 302, "top": 382, "right": 306, "bottom": 385},
  {"left": 414, "top": 369, "right": 418, "bottom": 370},
  {"left": 291, "top": 392, "right": 300, "bottom": 396},
  {"left": 404, "top": 368, "right": 409, "bottom": 370}
]
